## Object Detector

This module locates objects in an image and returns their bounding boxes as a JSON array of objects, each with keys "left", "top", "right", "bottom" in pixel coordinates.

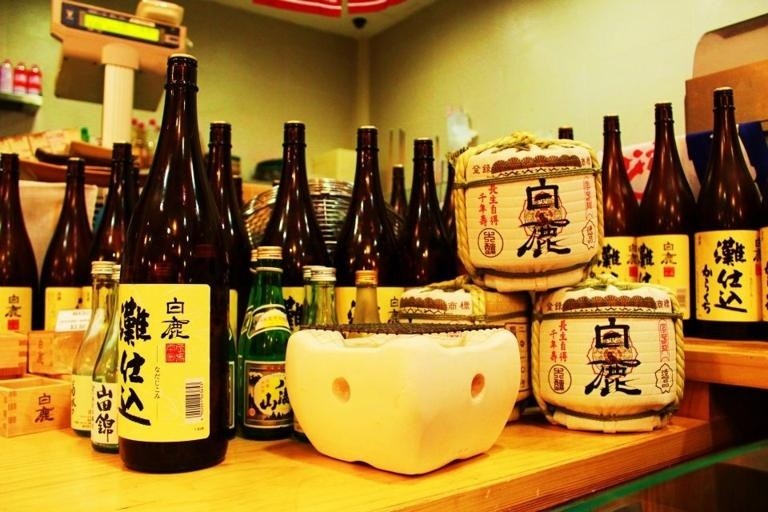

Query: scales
[{"left": 50, "top": 0, "right": 187, "bottom": 152}]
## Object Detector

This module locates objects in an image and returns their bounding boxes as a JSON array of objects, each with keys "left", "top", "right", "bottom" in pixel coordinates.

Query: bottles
[
  {"left": 0, "top": 51, "right": 460, "bottom": 474},
  {"left": 599, "top": 84, "right": 768, "bottom": 342}
]
